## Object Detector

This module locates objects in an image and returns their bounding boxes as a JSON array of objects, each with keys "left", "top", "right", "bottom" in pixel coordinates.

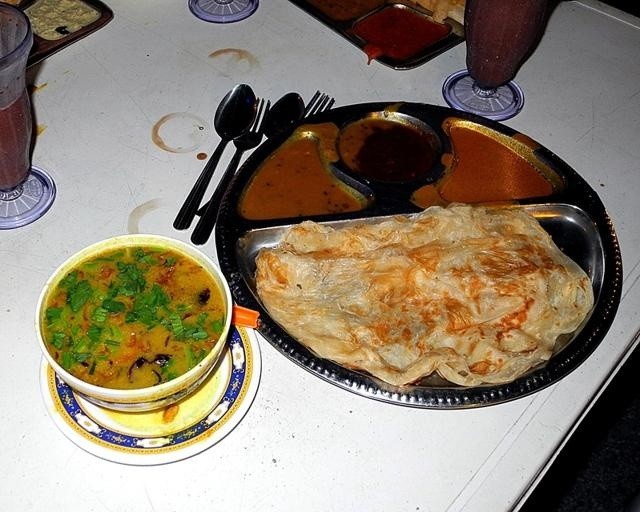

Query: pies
[{"left": 254, "top": 201, "right": 597, "bottom": 390}]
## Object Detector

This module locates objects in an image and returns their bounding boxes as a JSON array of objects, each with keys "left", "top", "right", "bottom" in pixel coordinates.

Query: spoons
[
  {"left": 196, "top": 91, "right": 306, "bottom": 218},
  {"left": 172, "top": 82, "right": 258, "bottom": 231}
]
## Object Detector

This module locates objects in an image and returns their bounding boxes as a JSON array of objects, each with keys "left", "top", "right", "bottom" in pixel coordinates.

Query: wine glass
[
  {"left": 441, "top": 0, "right": 566, "bottom": 122},
  {"left": 0, "top": 2, "right": 59, "bottom": 232}
]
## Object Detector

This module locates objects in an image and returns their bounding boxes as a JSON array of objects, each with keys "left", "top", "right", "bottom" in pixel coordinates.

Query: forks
[
  {"left": 189, "top": 96, "right": 270, "bottom": 246},
  {"left": 303, "top": 89, "right": 336, "bottom": 118}
]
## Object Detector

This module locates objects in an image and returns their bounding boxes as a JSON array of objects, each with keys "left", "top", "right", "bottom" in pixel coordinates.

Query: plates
[
  {"left": 214, "top": 100, "right": 624, "bottom": 409},
  {"left": 37, "top": 328, "right": 263, "bottom": 467}
]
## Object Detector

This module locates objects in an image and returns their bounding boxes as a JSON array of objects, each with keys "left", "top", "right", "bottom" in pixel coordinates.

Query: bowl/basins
[{"left": 32, "top": 233, "right": 234, "bottom": 415}]
